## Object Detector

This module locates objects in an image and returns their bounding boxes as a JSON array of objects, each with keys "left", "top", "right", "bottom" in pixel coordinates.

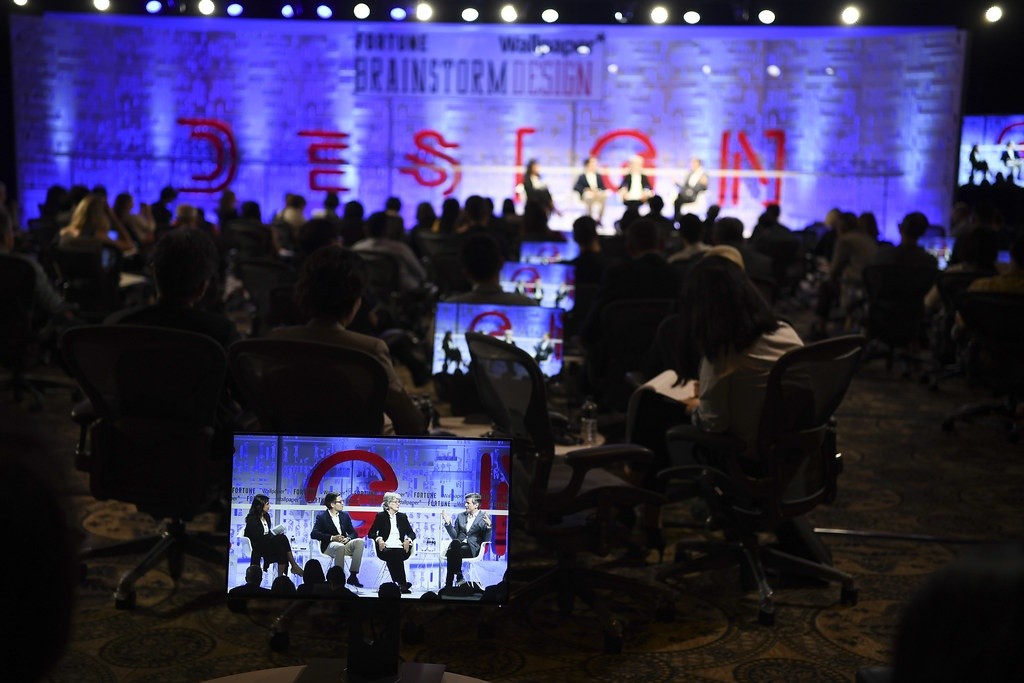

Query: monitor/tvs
[
  {"left": 498, "top": 260, "right": 578, "bottom": 312},
  {"left": 425, "top": 296, "right": 568, "bottom": 384},
  {"left": 518, "top": 240, "right": 580, "bottom": 264},
  {"left": 959, "top": 114, "right": 1024, "bottom": 188},
  {"left": 218, "top": 427, "right": 512, "bottom": 613}
]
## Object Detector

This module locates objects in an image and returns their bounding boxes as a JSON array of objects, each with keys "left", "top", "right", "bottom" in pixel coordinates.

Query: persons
[
  {"left": 2, "top": 151, "right": 1024, "bottom": 504},
  {"left": 1001, "top": 141, "right": 1021, "bottom": 179},
  {"left": 229, "top": 564, "right": 273, "bottom": 599},
  {"left": 268, "top": 573, "right": 297, "bottom": 599},
  {"left": 376, "top": 581, "right": 404, "bottom": 599},
  {"left": 367, "top": 496, "right": 417, "bottom": 592},
  {"left": 417, "top": 565, "right": 507, "bottom": 598},
  {"left": 967, "top": 142, "right": 989, "bottom": 184},
  {"left": 441, "top": 491, "right": 491, "bottom": 593},
  {"left": 311, "top": 491, "right": 366, "bottom": 588},
  {"left": 0, "top": 419, "right": 83, "bottom": 681},
  {"left": 681, "top": 255, "right": 841, "bottom": 584},
  {"left": 296, "top": 556, "right": 334, "bottom": 598},
  {"left": 245, "top": 492, "right": 306, "bottom": 580},
  {"left": 324, "top": 563, "right": 360, "bottom": 600}
]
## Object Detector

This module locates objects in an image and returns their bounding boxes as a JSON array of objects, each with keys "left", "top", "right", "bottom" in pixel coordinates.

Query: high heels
[{"left": 290, "top": 568, "right": 304, "bottom": 577}]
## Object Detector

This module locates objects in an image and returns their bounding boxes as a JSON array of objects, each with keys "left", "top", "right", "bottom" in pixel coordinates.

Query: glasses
[
  {"left": 335, "top": 500, "right": 345, "bottom": 504},
  {"left": 389, "top": 500, "right": 400, "bottom": 504}
]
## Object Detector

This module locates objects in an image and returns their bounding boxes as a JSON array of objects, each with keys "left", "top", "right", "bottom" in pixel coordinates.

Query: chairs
[{"left": 0, "top": 219, "right": 1024, "bottom": 633}]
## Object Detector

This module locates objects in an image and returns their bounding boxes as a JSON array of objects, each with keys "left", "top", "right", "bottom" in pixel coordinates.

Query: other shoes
[
  {"left": 455, "top": 574, "right": 465, "bottom": 585},
  {"left": 399, "top": 582, "right": 412, "bottom": 594},
  {"left": 347, "top": 576, "right": 364, "bottom": 588}
]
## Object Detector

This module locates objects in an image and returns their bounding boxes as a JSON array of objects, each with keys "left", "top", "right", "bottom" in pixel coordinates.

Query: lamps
[{"left": 15, "top": 0, "right": 862, "bottom": 28}]
